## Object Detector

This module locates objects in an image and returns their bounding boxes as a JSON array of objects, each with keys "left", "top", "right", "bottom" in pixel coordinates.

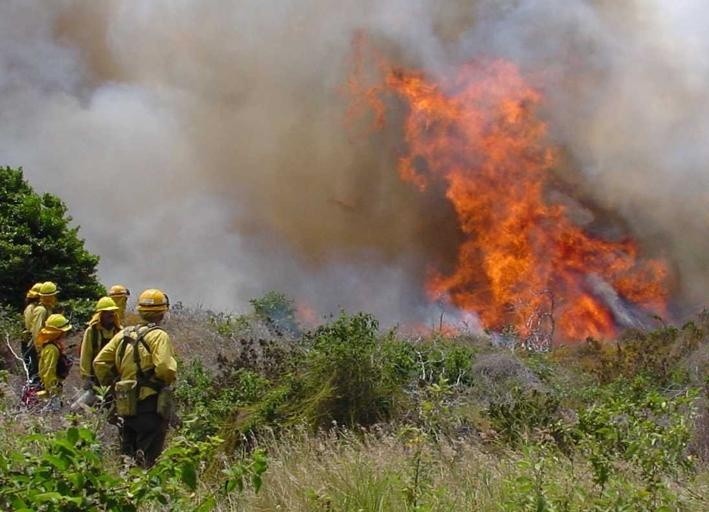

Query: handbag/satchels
[
  {"left": 113, "top": 378, "right": 139, "bottom": 418},
  {"left": 155, "top": 386, "right": 176, "bottom": 422}
]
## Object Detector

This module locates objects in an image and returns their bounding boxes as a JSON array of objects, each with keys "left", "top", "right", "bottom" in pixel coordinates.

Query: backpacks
[{"left": 51, "top": 341, "right": 73, "bottom": 379}]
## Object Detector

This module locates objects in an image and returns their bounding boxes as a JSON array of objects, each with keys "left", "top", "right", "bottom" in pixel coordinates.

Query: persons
[
  {"left": 106, "top": 283, "right": 130, "bottom": 327},
  {"left": 29, "top": 281, "right": 61, "bottom": 389},
  {"left": 74, "top": 295, "right": 124, "bottom": 429},
  {"left": 20, "top": 282, "right": 43, "bottom": 386},
  {"left": 91, "top": 288, "right": 177, "bottom": 469},
  {"left": 36, "top": 312, "right": 73, "bottom": 410}
]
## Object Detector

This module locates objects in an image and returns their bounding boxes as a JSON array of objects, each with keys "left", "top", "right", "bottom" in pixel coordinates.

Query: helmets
[
  {"left": 38, "top": 281, "right": 61, "bottom": 297},
  {"left": 95, "top": 296, "right": 120, "bottom": 312},
  {"left": 26, "top": 282, "right": 45, "bottom": 299},
  {"left": 108, "top": 284, "right": 130, "bottom": 298},
  {"left": 134, "top": 288, "right": 169, "bottom": 313},
  {"left": 40, "top": 313, "right": 73, "bottom": 335}
]
